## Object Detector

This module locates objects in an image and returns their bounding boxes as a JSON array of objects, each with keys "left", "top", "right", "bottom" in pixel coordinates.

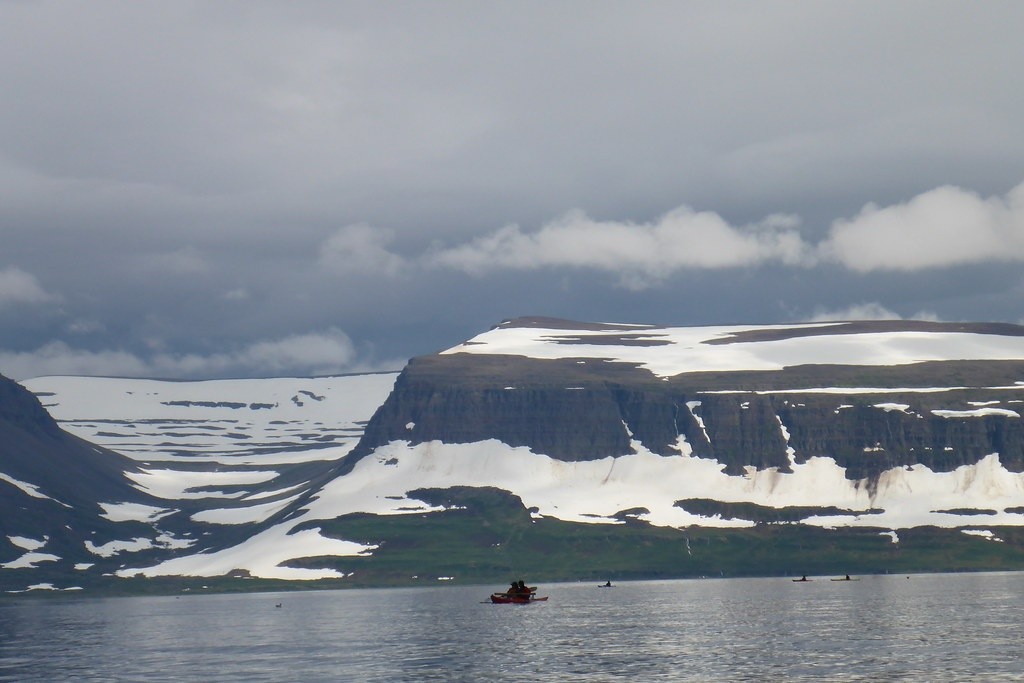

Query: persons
[
  {"left": 508, "top": 580, "right": 531, "bottom": 598},
  {"left": 846, "top": 574, "right": 850, "bottom": 579},
  {"left": 605, "top": 580, "right": 612, "bottom": 587},
  {"left": 802, "top": 575, "right": 806, "bottom": 580}
]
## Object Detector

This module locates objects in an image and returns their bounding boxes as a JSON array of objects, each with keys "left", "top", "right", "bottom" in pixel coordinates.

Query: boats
[
  {"left": 831, "top": 579, "right": 860, "bottom": 581},
  {"left": 491, "top": 594, "right": 529, "bottom": 604},
  {"left": 597, "top": 585, "right": 616, "bottom": 588},
  {"left": 793, "top": 579, "right": 813, "bottom": 583}
]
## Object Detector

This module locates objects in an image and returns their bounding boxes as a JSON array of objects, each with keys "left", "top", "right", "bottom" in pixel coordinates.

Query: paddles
[{"left": 494, "top": 586, "right": 537, "bottom": 596}]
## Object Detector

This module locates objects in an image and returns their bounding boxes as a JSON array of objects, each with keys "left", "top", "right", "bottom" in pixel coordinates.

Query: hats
[{"left": 510, "top": 582, "right": 519, "bottom": 585}]
